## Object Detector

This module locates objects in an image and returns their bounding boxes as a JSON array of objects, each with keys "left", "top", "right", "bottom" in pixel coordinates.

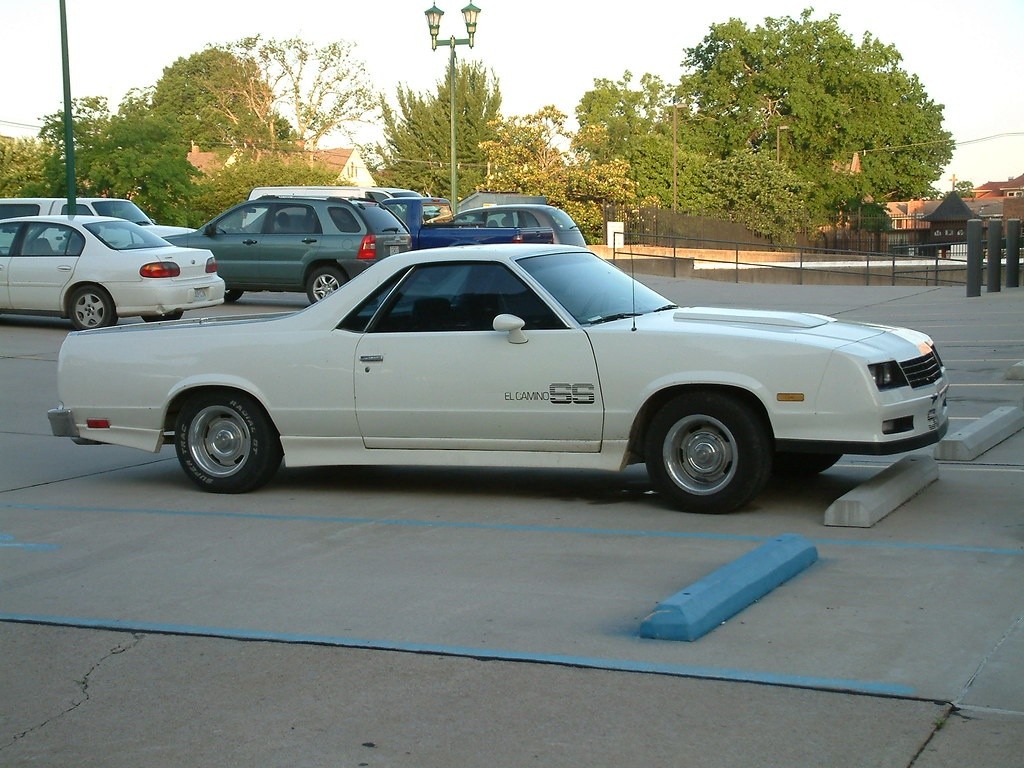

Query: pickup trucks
[
  {"left": 380, "top": 195, "right": 555, "bottom": 254},
  {"left": 1, "top": 197, "right": 200, "bottom": 247}
]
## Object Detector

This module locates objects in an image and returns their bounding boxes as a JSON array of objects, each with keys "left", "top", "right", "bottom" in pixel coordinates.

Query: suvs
[{"left": 125, "top": 193, "right": 417, "bottom": 306}]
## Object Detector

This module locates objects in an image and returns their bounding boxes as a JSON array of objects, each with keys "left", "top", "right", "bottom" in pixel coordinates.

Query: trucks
[{"left": 240, "top": 187, "right": 439, "bottom": 250}]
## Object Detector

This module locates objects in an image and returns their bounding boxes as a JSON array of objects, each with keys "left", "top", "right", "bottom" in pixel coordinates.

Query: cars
[
  {"left": 45, "top": 243, "right": 949, "bottom": 517},
  {"left": 0, "top": 215, "right": 226, "bottom": 334}
]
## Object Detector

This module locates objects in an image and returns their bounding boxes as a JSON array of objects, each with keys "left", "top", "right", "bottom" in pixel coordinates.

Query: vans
[{"left": 439, "top": 203, "right": 587, "bottom": 251}]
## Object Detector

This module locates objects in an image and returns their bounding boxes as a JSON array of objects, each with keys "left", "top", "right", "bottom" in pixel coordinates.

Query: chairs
[
  {"left": 501, "top": 216, "right": 514, "bottom": 227},
  {"left": 70, "top": 237, "right": 81, "bottom": 253},
  {"left": 30, "top": 238, "right": 54, "bottom": 254},
  {"left": 278, "top": 212, "right": 290, "bottom": 231},
  {"left": 488, "top": 220, "right": 498, "bottom": 227},
  {"left": 412, "top": 297, "right": 459, "bottom": 330}
]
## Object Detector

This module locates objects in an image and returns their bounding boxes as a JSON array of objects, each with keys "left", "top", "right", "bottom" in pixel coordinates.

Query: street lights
[{"left": 425, "top": 0, "right": 480, "bottom": 217}]
